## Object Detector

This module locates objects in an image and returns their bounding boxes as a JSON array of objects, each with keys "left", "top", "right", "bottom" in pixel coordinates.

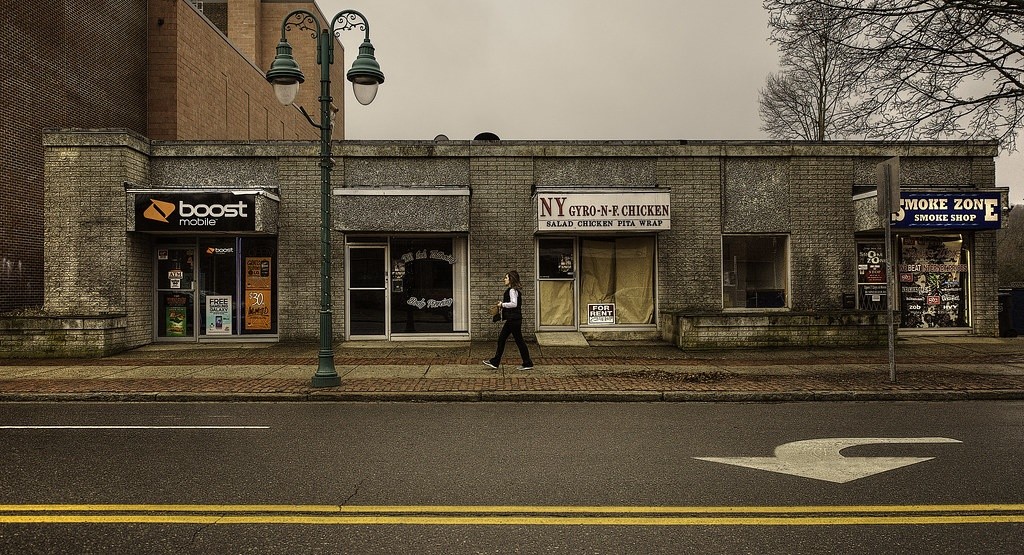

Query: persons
[{"left": 482, "top": 271, "right": 534, "bottom": 371}]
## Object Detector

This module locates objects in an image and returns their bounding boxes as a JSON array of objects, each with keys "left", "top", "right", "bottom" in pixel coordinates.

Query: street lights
[{"left": 266, "top": 7, "right": 386, "bottom": 387}]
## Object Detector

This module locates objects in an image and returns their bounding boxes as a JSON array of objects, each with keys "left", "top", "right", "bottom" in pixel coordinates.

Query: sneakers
[
  {"left": 517, "top": 366, "right": 534, "bottom": 370},
  {"left": 482, "top": 360, "right": 497, "bottom": 369}
]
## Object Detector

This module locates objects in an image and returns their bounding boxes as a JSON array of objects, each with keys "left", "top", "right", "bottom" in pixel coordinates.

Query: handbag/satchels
[{"left": 493, "top": 306, "right": 501, "bottom": 322}]
